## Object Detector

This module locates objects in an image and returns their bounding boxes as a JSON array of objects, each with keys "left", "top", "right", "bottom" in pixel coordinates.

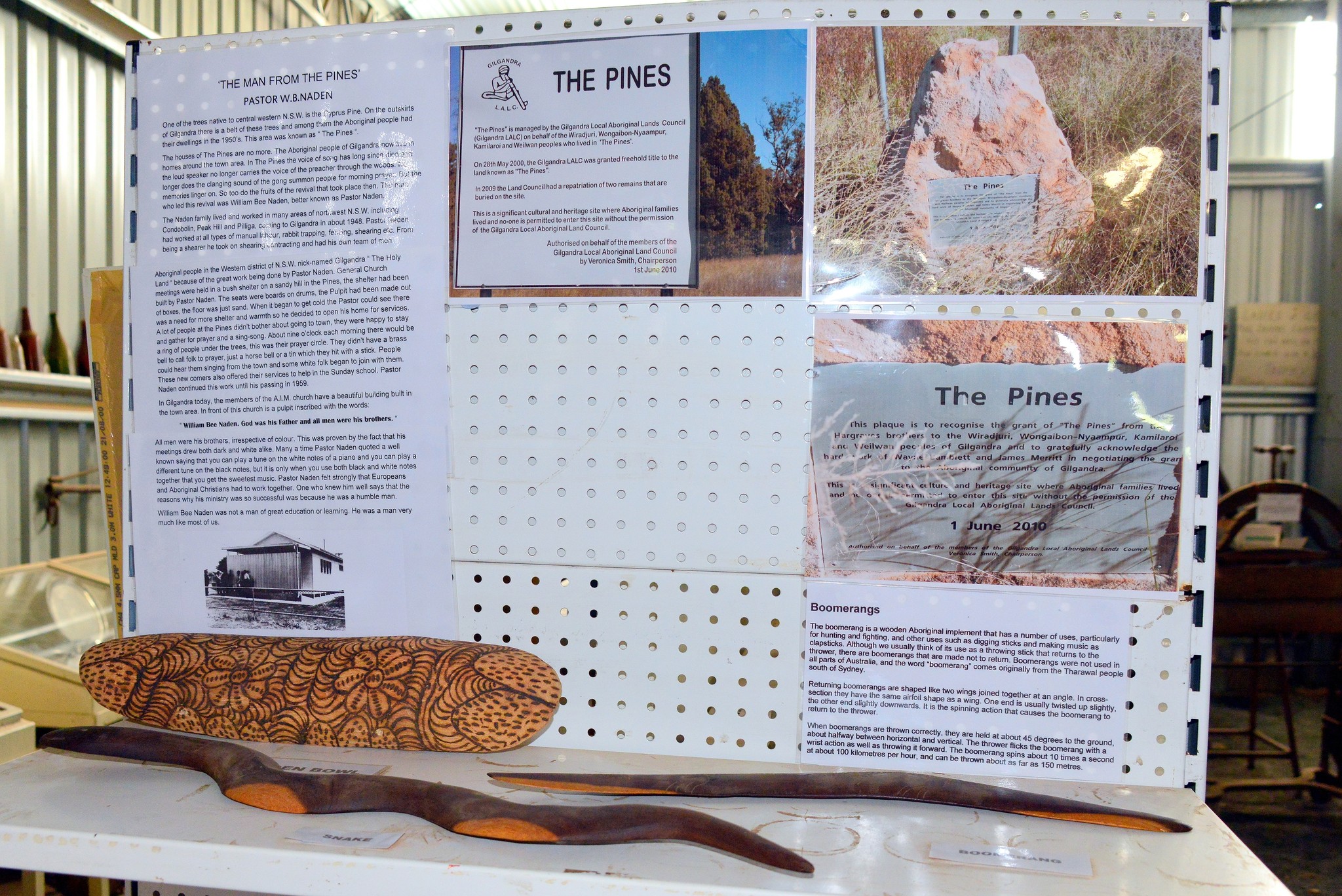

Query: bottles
[
  {"left": 49, "top": 310, "right": 69, "bottom": 373},
  {"left": 1, "top": 327, "right": 8, "bottom": 368},
  {"left": 77, "top": 319, "right": 91, "bottom": 375},
  {"left": 20, "top": 306, "right": 39, "bottom": 370}
]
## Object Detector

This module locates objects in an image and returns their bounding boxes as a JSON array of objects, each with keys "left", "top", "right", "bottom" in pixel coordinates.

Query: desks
[{"left": 0, "top": 712, "right": 1304, "bottom": 896}]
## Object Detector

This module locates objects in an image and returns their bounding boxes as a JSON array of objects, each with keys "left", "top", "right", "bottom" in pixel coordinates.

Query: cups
[{"left": 1253, "top": 445, "right": 1298, "bottom": 483}]
